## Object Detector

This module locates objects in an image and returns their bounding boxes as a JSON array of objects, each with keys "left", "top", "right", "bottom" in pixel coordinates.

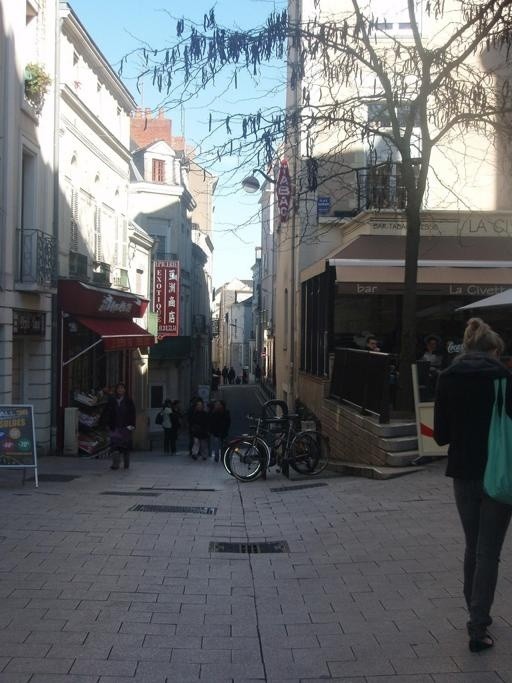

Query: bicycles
[{"left": 221, "top": 398, "right": 332, "bottom": 483}]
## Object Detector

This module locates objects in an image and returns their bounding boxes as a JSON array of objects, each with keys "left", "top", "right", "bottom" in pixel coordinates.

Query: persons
[
  {"left": 160, "top": 398, "right": 179, "bottom": 457},
  {"left": 104, "top": 381, "right": 136, "bottom": 469},
  {"left": 208, "top": 399, "right": 231, "bottom": 461},
  {"left": 429, "top": 316, "right": 512, "bottom": 653},
  {"left": 222, "top": 366, "right": 229, "bottom": 384},
  {"left": 228, "top": 366, "right": 236, "bottom": 384},
  {"left": 417, "top": 331, "right": 452, "bottom": 370},
  {"left": 216, "top": 368, "right": 222, "bottom": 376},
  {"left": 189, "top": 402, "right": 212, "bottom": 460},
  {"left": 362, "top": 336, "right": 383, "bottom": 352}
]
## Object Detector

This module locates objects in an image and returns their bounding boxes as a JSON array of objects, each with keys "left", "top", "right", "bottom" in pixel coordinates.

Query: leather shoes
[{"left": 469, "top": 635, "right": 493, "bottom": 652}]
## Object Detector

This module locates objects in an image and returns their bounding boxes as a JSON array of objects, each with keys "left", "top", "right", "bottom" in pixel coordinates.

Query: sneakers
[
  {"left": 124, "top": 462, "right": 129, "bottom": 468},
  {"left": 110, "top": 464, "right": 119, "bottom": 469}
]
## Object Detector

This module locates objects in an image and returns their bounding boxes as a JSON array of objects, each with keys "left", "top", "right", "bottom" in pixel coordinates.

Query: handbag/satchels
[
  {"left": 484, "top": 405, "right": 512, "bottom": 505},
  {"left": 155, "top": 412, "right": 164, "bottom": 425}
]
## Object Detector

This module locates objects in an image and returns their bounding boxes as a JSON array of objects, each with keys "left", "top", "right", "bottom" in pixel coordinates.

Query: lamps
[{"left": 242, "top": 168, "right": 275, "bottom": 194}]
